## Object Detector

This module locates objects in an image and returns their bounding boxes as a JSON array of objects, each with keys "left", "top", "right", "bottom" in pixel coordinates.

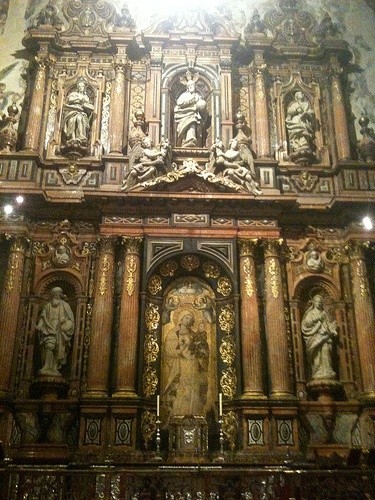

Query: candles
[
  {"left": 219, "top": 393, "right": 223, "bottom": 416},
  {"left": 156, "top": 394, "right": 160, "bottom": 416}
]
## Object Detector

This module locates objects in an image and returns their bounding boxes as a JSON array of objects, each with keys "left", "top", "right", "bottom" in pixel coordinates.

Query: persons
[
  {"left": 307, "top": 250, "right": 324, "bottom": 270},
  {"left": 61, "top": 82, "right": 95, "bottom": 147},
  {"left": 284, "top": 91, "right": 314, "bottom": 153},
  {"left": 173, "top": 80, "right": 206, "bottom": 143},
  {"left": 35, "top": 287, "right": 75, "bottom": 375},
  {"left": 215, "top": 138, "right": 263, "bottom": 196},
  {"left": 120, "top": 137, "right": 168, "bottom": 192},
  {"left": 54, "top": 245, "right": 69, "bottom": 264},
  {"left": 162, "top": 309, "right": 208, "bottom": 416},
  {"left": 189, "top": 320, "right": 210, "bottom": 359},
  {"left": 300, "top": 294, "right": 339, "bottom": 380}
]
